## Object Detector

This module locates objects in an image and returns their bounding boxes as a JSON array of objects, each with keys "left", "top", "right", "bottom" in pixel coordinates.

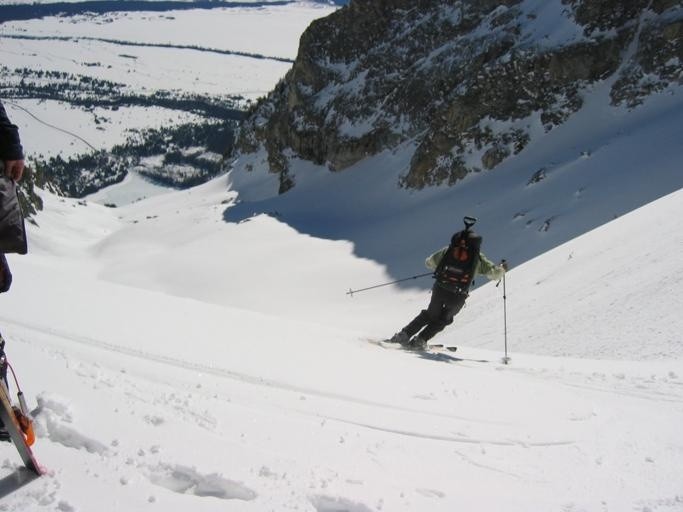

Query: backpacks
[{"left": 434, "top": 230, "right": 481, "bottom": 294}]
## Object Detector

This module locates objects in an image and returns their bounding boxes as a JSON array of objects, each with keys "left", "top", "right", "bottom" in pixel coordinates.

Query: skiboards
[
  {"left": 0, "top": 378, "right": 46, "bottom": 474},
  {"left": 359, "top": 337, "right": 457, "bottom": 353}
]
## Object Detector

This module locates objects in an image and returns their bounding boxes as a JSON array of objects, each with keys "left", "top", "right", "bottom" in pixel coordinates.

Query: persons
[
  {"left": 0, "top": 98, "right": 27, "bottom": 439},
  {"left": 386, "top": 227, "right": 508, "bottom": 353}
]
